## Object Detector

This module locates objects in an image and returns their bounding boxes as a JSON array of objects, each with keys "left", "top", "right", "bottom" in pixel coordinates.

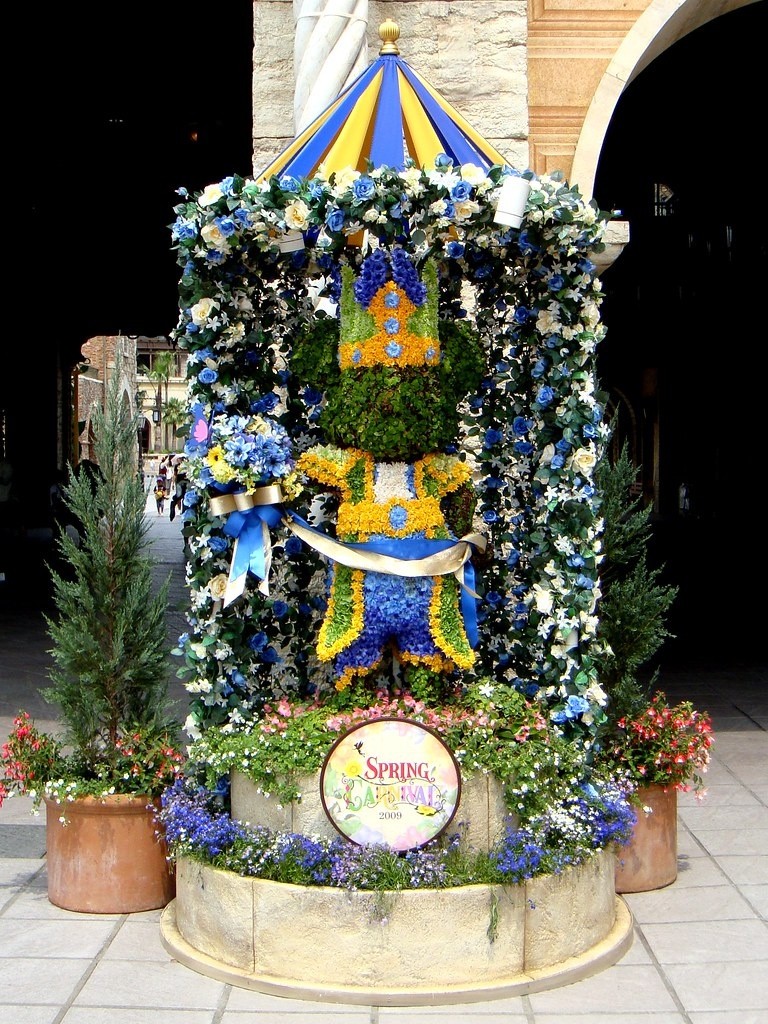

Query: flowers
[
  {"left": 0, "top": 336, "right": 192, "bottom": 827},
  {"left": 142, "top": 154, "right": 654, "bottom": 944},
  {"left": 598, "top": 691, "right": 718, "bottom": 802}
]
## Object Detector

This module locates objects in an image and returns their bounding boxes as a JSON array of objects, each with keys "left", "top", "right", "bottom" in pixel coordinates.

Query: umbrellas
[{"left": 171, "top": 453, "right": 189, "bottom": 466}]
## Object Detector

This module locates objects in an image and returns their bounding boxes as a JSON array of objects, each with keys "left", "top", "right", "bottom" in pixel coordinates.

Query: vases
[
  {"left": 613, "top": 781, "right": 680, "bottom": 895},
  {"left": 38, "top": 788, "right": 177, "bottom": 915}
]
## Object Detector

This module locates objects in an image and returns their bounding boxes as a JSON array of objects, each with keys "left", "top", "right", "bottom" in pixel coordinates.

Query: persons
[
  {"left": 153, "top": 477, "right": 167, "bottom": 517},
  {"left": 172, "top": 457, "right": 189, "bottom": 510},
  {"left": 159, "top": 456, "right": 174, "bottom": 500}
]
